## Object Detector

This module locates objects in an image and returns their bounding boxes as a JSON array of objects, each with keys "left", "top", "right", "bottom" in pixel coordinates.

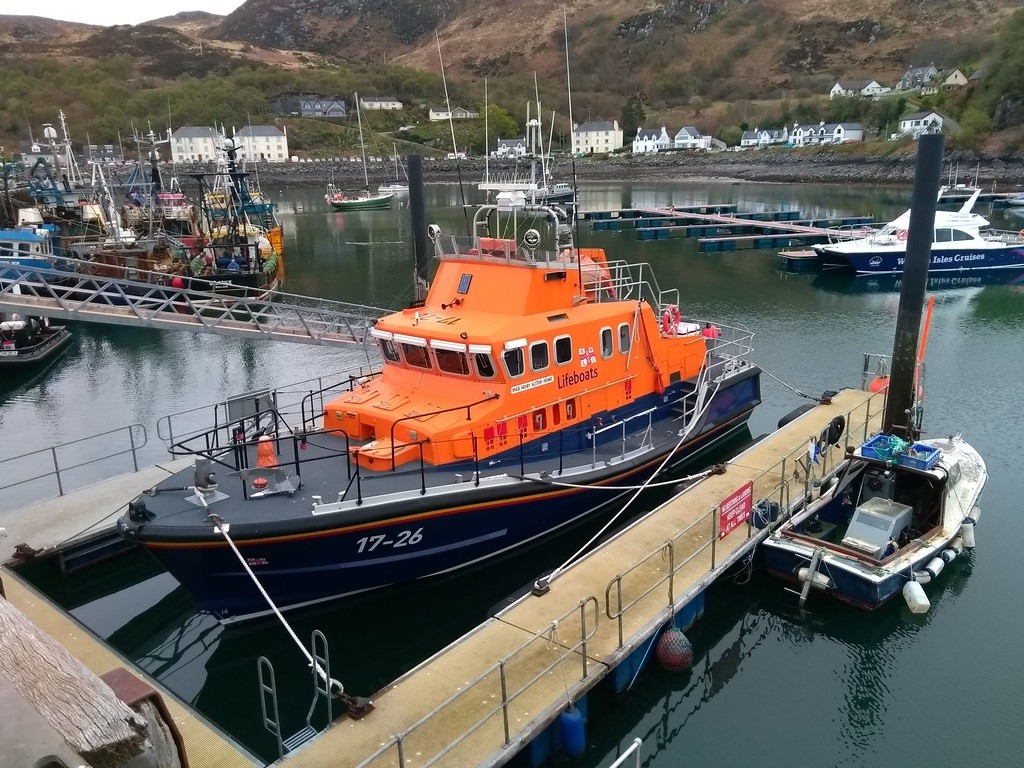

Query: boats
[
  {"left": 808, "top": 159, "right": 1024, "bottom": 294},
  {"left": 754, "top": 356, "right": 990, "bottom": 617},
  {"left": 114, "top": 9, "right": 766, "bottom": 633},
  {"left": 0, "top": 312, "right": 74, "bottom": 369}
]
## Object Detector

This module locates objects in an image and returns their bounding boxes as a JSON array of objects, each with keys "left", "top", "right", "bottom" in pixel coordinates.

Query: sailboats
[
  {"left": 324, "top": 91, "right": 398, "bottom": 211},
  {"left": 0, "top": 95, "right": 284, "bottom": 324},
  {"left": 524, "top": 112, "right": 580, "bottom": 207},
  {"left": 377, "top": 143, "right": 410, "bottom": 198}
]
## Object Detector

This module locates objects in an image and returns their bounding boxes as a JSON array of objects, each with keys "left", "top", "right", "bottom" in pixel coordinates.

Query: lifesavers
[
  {"left": 1019, "top": 228, "right": 1024, "bottom": 239},
  {"left": 897, "top": 229, "right": 908, "bottom": 241},
  {"left": 663, "top": 305, "right": 680, "bottom": 335}
]
